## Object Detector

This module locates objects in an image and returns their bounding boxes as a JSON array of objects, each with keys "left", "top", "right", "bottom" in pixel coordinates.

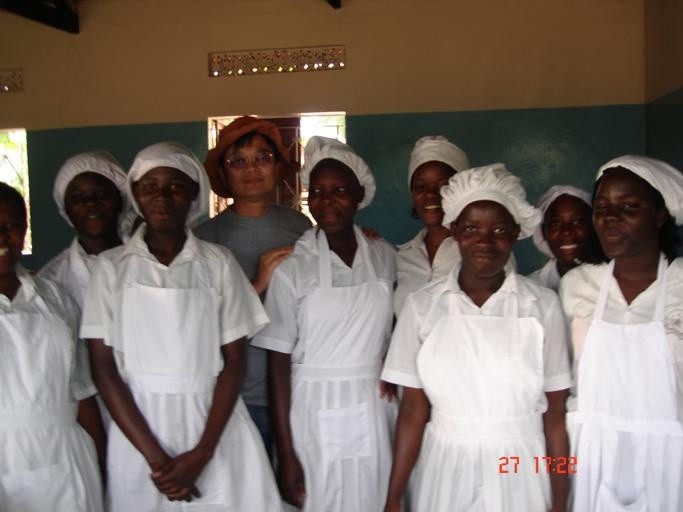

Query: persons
[
  {"left": 80, "top": 141, "right": 281, "bottom": 511},
  {"left": 37, "top": 149, "right": 134, "bottom": 421},
  {"left": 247, "top": 135, "right": 397, "bottom": 510},
  {"left": 191, "top": 113, "right": 378, "bottom": 469},
  {"left": 519, "top": 182, "right": 593, "bottom": 292},
  {"left": 394, "top": 132, "right": 517, "bottom": 314},
  {"left": 377, "top": 164, "right": 572, "bottom": 511},
  {"left": 559, "top": 153, "right": 682, "bottom": 511},
  {"left": 2, "top": 178, "right": 104, "bottom": 510}
]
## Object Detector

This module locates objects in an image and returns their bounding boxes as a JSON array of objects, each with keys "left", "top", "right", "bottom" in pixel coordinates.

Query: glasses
[{"left": 226, "top": 153, "right": 274, "bottom": 166}]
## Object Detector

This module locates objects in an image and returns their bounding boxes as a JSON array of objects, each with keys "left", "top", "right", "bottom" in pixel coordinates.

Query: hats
[
  {"left": 52, "top": 149, "right": 128, "bottom": 227},
  {"left": 297, "top": 136, "right": 376, "bottom": 210},
  {"left": 205, "top": 116, "right": 300, "bottom": 198},
  {"left": 440, "top": 163, "right": 541, "bottom": 240}
]
